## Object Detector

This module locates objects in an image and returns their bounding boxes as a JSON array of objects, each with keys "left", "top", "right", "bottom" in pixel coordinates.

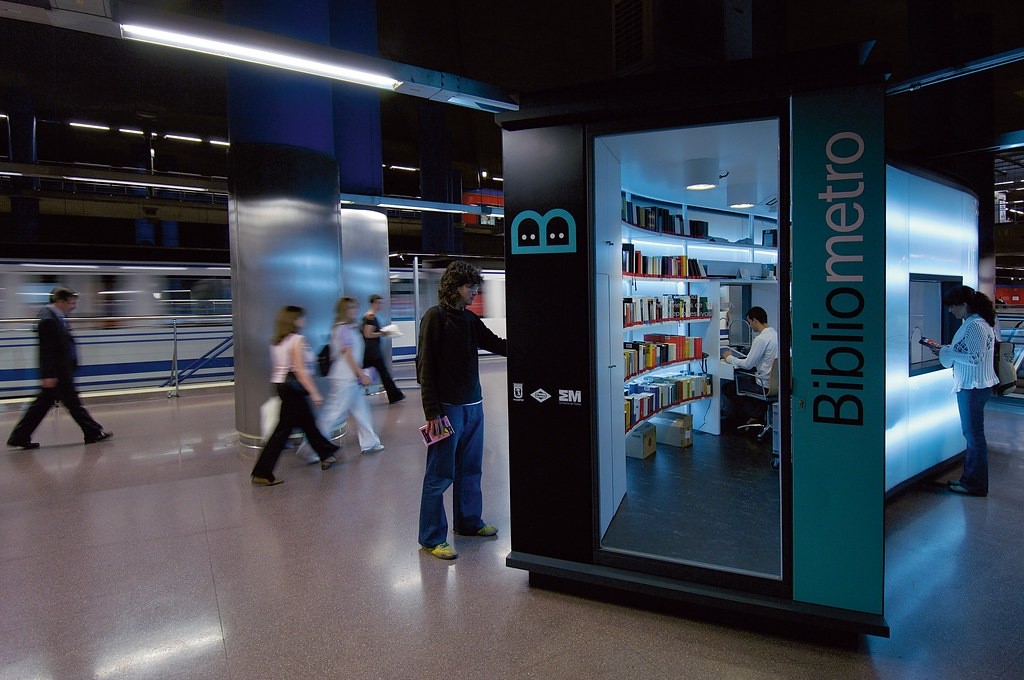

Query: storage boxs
[
  {"left": 648, "top": 412, "right": 693, "bottom": 447},
  {"left": 626, "top": 421, "right": 656, "bottom": 459}
]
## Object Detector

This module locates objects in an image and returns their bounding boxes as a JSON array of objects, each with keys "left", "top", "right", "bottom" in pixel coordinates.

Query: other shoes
[
  {"left": 947, "top": 479, "right": 976, "bottom": 495},
  {"left": 362, "top": 444, "right": 385, "bottom": 453},
  {"left": 83, "top": 431, "right": 114, "bottom": 443},
  {"left": 296, "top": 447, "right": 320, "bottom": 463},
  {"left": 6, "top": 438, "right": 41, "bottom": 449},
  {"left": 251, "top": 474, "right": 284, "bottom": 487},
  {"left": 745, "top": 417, "right": 759, "bottom": 431},
  {"left": 321, "top": 454, "right": 343, "bottom": 470}
]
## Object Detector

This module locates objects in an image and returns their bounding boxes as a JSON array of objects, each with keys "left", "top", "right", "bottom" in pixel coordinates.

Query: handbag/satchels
[
  {"left": 993, "top": 338, "right": 1017, "bottom": 397},
  {"left": 258, "top": 396, "right": 285, "bottom": 442}
]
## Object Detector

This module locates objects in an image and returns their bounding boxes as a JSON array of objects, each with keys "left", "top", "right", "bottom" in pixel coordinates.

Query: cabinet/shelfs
[{"left": 621, "top": 187, "right": 778, "bottom": 437}]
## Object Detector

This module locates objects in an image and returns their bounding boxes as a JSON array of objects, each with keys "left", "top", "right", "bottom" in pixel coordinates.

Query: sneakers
[
  {"left": 421, "top": 540, "right": 457, "bottom": 559},
  {"left": 454, "top": 524, "right": 500, "bottom": 536}
]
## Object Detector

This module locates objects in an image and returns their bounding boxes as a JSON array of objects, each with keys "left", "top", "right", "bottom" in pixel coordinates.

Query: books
[
  {"left": 624, "top": 370, "right": 713, "bottom": 435},
  {"left": 622, "top": 243, "right": 706, "bottom": 279},
  {"left": 419, "top": 416, "right": 455, "bottom": 446},
  {"left": 622, "top": 333, "right": 703, "bottom": 382},
  {"left": 919, "top": 337, "right": 940, "bottom": 349},
  {"left": 623, "top": 294, "right": 712, "bottom": 327},
  {"left": 620, "top": 191, "right": 709, "bottom": 238}
]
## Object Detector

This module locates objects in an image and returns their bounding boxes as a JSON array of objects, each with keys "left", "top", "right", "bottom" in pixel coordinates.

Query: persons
[
  {"left": 722, "top": 306, "right": 778, "bottom": 431},
  {"left": 415, "top": 260, "right": 507, "bottom": 559},
  {"left": 930, "top": 285, "right": 1001, "bottom": 497},
  {"left": 250, "top": 305, "right": 340, "bottom": 487},
  {"left": 359, "top": 295, "right": 407, "bottom": 404},
  {"left": 295, "top": 297, "right": 384, "bottom": 463},
  {"left": 6, "top": 287, "right": 114, "bottom": 448}
]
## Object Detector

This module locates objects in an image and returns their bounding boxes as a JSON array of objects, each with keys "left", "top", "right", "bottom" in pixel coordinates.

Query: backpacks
[{"left": 318, "top": 324, "right": 344, "bottom": 377}]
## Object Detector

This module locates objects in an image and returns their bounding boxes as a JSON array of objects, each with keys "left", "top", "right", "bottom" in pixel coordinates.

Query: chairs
[{"left": 736, "top": 357, "right": 792, "bottom": 439}]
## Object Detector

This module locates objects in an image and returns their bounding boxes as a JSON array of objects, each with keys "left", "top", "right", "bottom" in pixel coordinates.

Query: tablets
[{"left": 719, "top": 346, "right": 746, "bottom": 359}]
